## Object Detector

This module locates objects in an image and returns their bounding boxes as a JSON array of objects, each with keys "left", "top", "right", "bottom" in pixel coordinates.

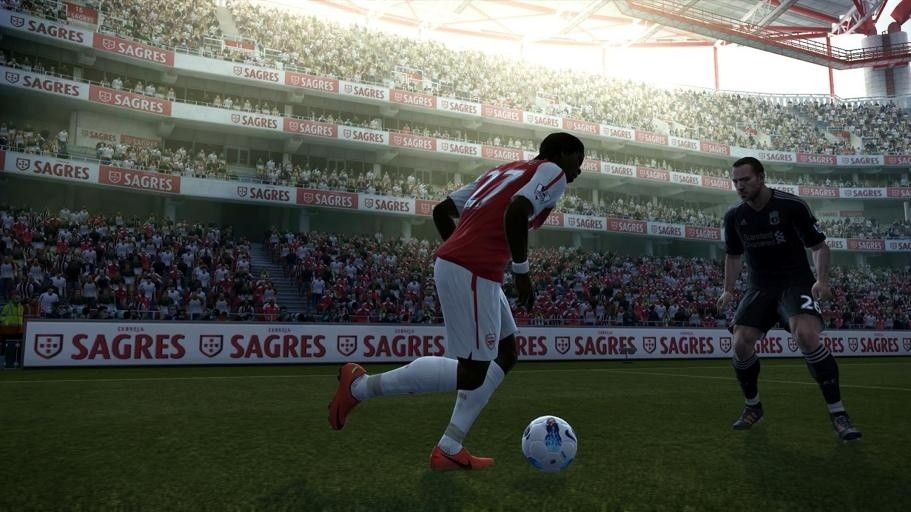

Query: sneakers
[
  {"left": 732, "top": 401, "right": 764, "bottom": 431},
  {"left": 828, "top": 411, "right": 862, "bottom": 440},
  {"left": 327, "top": 363, "right": 366, "bottom": 431},
  {"left": 429, "top": 445, "right": 493, "bottom": 472}
]
